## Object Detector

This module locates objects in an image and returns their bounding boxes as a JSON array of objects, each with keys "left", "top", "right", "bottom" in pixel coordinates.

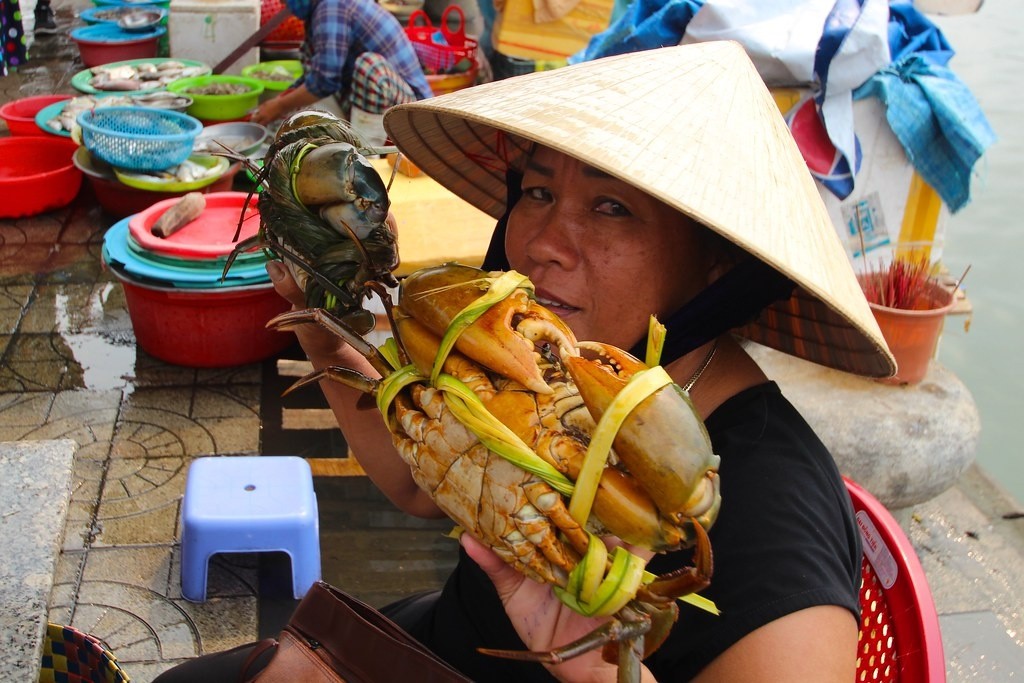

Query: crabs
[
  {"left": 266, "top": 259, "right": 721, "bottom": 683},
  {"left": 205, "top": 109, "right": 399, "bottom": 318}
]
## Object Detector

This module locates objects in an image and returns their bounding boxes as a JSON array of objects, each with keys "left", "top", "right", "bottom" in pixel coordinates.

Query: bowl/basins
[
  {"left": 87, "top": 159, "right": 242, "bottom": 217},
  {"left": 67, "top": 0, "right": 171, "bottom": 67},
  {"left": 73, "top": 145, "right": 114, "bottom": 200},
  {"left": 0, "top": 94, "right": 76, "bottom": 136},
  {"left": 192, "top": 121, "right": 267, "bottom": 156},
  {"left": 167, "top": 74, "right": 264, "bottom": 121},
  {"left": 0, "top": 136, "right": 83, "bottom": 219},
  {"left": 117, "top": 12, "right": 163, "bottom": 31},
  {"left": 241, "top": 60, "right": 303, "bottom": 91},
  {"left": 100, "top": 254, "right": 298, "bottom": 369}
]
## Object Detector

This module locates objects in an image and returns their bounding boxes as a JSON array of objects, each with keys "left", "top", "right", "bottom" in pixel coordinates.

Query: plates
[{"left": 71, "top": 58, "right": 212, "bottom": 95}]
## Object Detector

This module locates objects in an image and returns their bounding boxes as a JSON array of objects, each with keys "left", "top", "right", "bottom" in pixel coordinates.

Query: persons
[
  {"left": 250, "top": 0, "right": 435, "bottom": 158},
  {"left": 151, "top": 39, "right": 898, "bottom": 683}
]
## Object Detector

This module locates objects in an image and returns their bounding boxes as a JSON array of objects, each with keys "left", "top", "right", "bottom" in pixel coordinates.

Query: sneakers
[{"left": 33, "top": 5, "right": 57, "bottom": 34}]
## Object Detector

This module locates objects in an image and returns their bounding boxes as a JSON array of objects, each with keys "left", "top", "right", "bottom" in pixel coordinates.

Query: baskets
[
  {"left": 402, "top": 5, "right": 479, "bottom": 75},
  {"left": 76, "top": 107, "right": 203, "bottom": 170}
]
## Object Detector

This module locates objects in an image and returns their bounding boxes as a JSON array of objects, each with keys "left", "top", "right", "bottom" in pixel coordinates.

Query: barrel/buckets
[{"left": 855, "top": 271, "right": 957, "bottom": 387}]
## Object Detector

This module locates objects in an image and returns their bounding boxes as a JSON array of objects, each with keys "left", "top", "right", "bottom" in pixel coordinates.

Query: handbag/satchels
[
  {"left": 239, "top": 582, "right": 476, "bottom": 683},
  {"left": 37, "top": 621, "right": 133, "bottom": 683}
]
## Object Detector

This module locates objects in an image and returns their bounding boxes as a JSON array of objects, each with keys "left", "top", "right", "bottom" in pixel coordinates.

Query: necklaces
[{"left": 682, "top": 345, "right": 718, "bottom": 392}]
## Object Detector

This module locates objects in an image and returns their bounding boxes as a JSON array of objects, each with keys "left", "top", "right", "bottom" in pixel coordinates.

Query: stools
[{"left": 183, "top": 456, "right": 325, "bottom": 598}]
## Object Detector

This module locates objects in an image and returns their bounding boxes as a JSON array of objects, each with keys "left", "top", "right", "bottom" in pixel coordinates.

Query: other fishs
[{"left": 46, "top": 60, "right": 258, "bottom": 185}]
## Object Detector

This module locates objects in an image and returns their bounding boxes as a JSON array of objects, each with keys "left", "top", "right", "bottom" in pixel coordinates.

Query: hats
[{"left": 382, "top": 40, "right": 897, "bottom": 380}]
